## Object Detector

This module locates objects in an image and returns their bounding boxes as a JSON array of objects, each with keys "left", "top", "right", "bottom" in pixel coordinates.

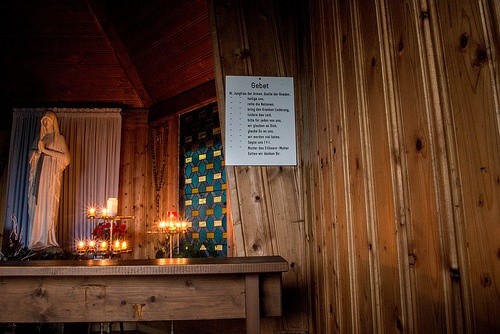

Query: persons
[{"left": 25, "top": 111, "right": 71, "bottom": 250}]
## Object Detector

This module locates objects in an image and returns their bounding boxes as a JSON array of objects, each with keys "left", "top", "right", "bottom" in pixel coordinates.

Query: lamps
[
  {"left": 157, "top": 211, "right": 189, "bottom": 258},
  {"left": 77, "top": 198, "right": 136, "bottom": 259}
]
